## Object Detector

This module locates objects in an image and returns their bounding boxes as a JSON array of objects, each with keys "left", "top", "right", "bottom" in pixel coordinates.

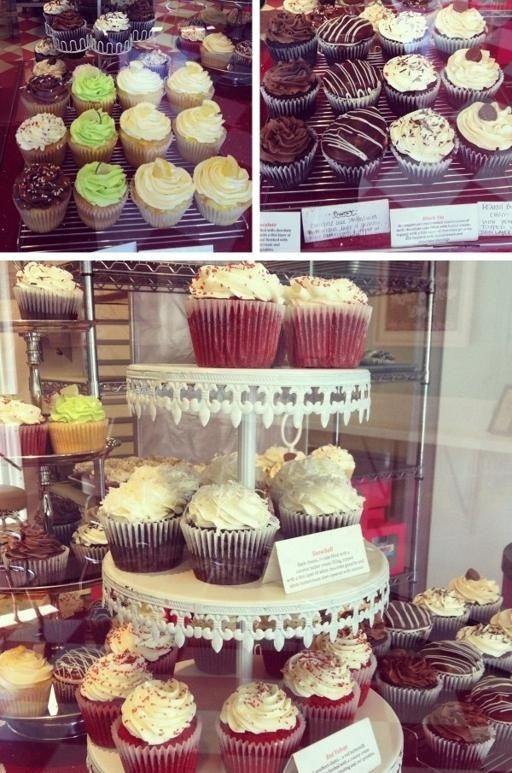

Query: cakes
[
  {"left": 13, "top": 0, "right": 252, "bottom": 230},
  {"left": 260, "top": 0, "right": 512, "bottom": 186},
  {"left": 0, "top": 261, "right": 512, "bottom": 773}
]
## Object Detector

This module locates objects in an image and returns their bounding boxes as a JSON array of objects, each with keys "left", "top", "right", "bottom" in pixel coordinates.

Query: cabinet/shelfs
[{"left": 27, "top": 261, "right": 452, "bottom": 606}]
